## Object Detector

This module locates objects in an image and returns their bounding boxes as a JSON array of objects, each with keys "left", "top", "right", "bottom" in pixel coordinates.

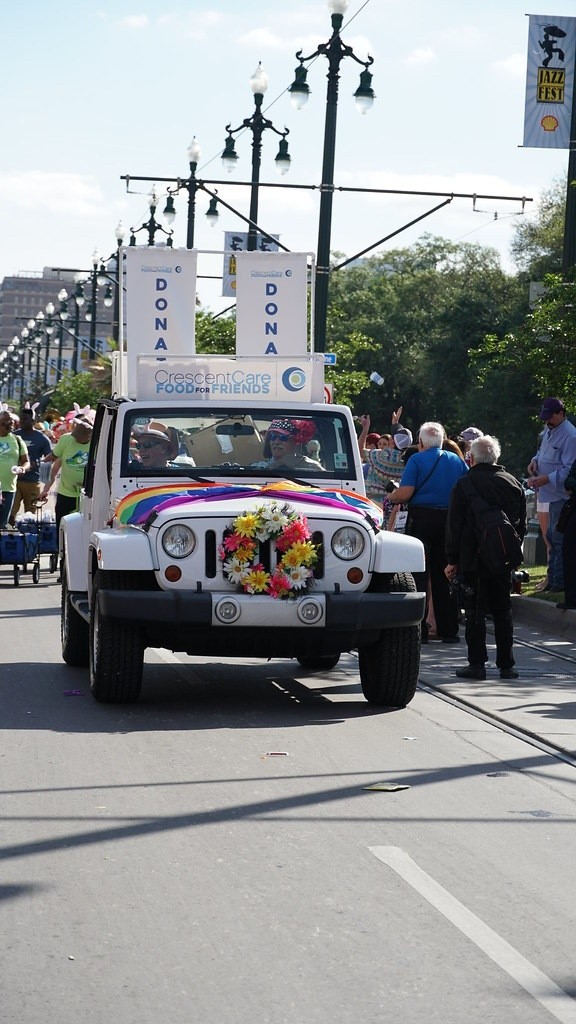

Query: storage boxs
[
  {"left": 185, "top": 415, "right": 265, "bottom": 467},
  {"left": 0, "top": 532, "right": 38, "bottom": 562},
  {"left": 17, "top": 524, "right": 58, "bottom": 552}
]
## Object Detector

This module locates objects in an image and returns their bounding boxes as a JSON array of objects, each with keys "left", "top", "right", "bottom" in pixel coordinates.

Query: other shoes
[
  {"left": 536, "top": 578, "right": 564, "bottom": 593},
  {"left": 555, "top": 600, "right": 576, "bottom": 609},
  {"left": 442, "top": 633, "right": 460, "bottom": 643}
]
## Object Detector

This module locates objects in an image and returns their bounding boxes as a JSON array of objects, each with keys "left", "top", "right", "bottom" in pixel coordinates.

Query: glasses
[
  {"left": 268, "top": 433, "right": 290, "bottom": 441},
  {"left": 136, "top": 441, "right": 160, "bottom": 450}
]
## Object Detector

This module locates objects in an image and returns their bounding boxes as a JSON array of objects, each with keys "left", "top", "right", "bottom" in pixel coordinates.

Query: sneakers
[
  {"left": 500, "top": 664, "right": 519, "bottom": 679},
  {"left": 456, "top": 662, "right": 486, "bottom": 680}
]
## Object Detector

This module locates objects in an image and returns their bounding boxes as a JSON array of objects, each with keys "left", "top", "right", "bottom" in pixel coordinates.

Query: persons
[
  {"left": 445, "top": 434, "right": 527, "bottom": 678},
  {"left": 556, "top": 459, "right": 576, "bottom": 609},
  {"left": 527, "top": 399, "right": 576, "bottom": 594},
  {"left": 130, "top": 422, "right": 179, "bottom": 467},
  {"left": 358, "top": 407, "right": 483, "bottom": 643},
  {"left": 0, "top": 402, "right": 96, "bottom": 583},
  {"left": 252, "top": 420, "right": 328, "bottom": 471}
]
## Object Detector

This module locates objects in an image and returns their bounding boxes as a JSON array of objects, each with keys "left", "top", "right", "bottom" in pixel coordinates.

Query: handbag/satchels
[{"left": 458, "top": 474, "right": 524, "bottom": 580}]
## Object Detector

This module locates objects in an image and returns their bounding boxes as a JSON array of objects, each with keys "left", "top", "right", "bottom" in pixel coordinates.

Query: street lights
[
  {"left": 99, "top": 220, "right": 128, "bottom": 351},
  {"left": 219, "top": 59, "right": 292, "bottom": 251},
  {"left": 73, "top": 246, "right": 114, "bottom": 361},
  {"left": 0, "top": 288, "right": 81, "bottom": 402},
  {"left": 130, "top": 193, "right": 175, "bottom": 251},
  {"left": 287, "top": 0, "right": 376, "bottom": 357},
  {"left": 160, "top": 135, "right": 220, "bottom": 251},
  {"left": 57, "top": 266, "right": 91, "bottom": 376}
]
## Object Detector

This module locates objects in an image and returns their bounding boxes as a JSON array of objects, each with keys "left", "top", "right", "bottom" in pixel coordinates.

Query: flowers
[
  {"left": 291, "top": 418, "right": 317, "bottom": 443},
  {"left": 218, "top": 503, "right": 320, "bottom": 600}
]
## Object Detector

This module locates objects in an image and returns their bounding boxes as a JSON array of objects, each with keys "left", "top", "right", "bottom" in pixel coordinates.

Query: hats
[
  {"left": 131, "top": 421, "right": 177, "bottom": 460},
  {"left": 539, "top": 398, "right": 565, "bottom": 421},
  {"left": 392, "top": 423, "right": 413, "bottom": 451},
  {"left": 366, "top": 431, "right": 381, "bottom": 448},
  {"left": 268, "top": 418, "right": 301, "bottom": 441},
  {"left": 73, "top": 410, "right": 96, "bottom": 430}
]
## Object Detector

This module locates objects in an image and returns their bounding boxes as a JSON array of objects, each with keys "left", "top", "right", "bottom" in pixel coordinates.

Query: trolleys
[{"left": 0, "top": 496, "right": 58, "bottom": 587}]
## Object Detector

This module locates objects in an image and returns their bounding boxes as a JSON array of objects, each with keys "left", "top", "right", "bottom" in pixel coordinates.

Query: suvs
[{"left": 56, "top": 391, "right": 428, "bottom": 711}]
